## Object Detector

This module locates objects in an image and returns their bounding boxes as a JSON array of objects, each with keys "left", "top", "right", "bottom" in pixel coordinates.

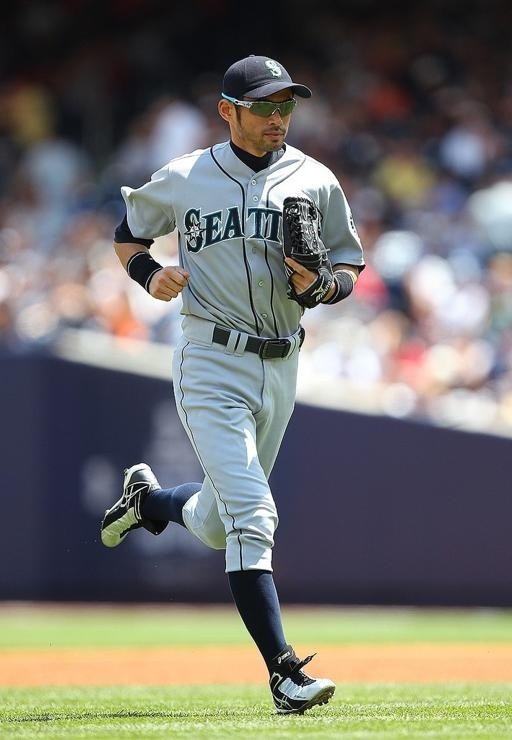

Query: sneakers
[
  {"left": 100, "top": 462, "right": 170, "bottom": 550},
  {"left": 267, "top": 644, "right": 337, "bottom": 716}
]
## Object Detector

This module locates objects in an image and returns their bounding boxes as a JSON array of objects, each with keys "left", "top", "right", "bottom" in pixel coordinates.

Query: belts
[{"left": 212, "top": 323, "right": 306, "bottom": 361}]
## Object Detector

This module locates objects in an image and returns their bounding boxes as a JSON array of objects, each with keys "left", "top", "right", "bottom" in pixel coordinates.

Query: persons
[
  {"left": 93, "top": 51, "right": 372, "bottom": 720},
  {"left": 0, "top": 94, "right": 512, "bottom": 428}
]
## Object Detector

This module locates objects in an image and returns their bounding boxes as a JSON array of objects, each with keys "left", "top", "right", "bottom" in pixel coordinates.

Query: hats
[{"left": 219, "top": 54, "right": 313, "bottom": 101}]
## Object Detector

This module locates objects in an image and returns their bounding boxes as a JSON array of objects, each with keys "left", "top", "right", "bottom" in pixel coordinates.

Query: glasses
[{"left": 220, "top": 93, "right": 297, "bottom": 119}]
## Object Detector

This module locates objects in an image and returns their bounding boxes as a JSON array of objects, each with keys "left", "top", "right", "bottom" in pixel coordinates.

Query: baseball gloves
[{"left": 280, "top": 196, "right": 329, "bottom": 273}]
